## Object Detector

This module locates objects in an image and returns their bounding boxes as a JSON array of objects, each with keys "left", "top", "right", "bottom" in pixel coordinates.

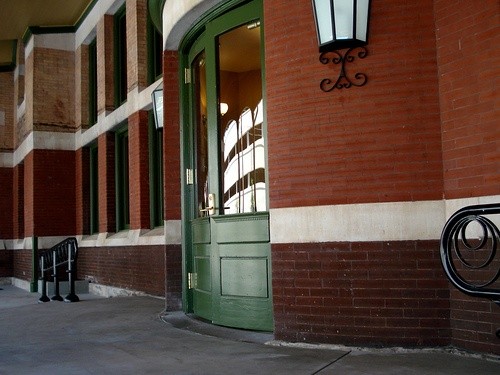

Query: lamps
[
  {"left": 312, "top": 0, "right": 372, "bottom": 92},
  {"left": 150, "top": 80, "right": 163, "bottom": 130}
]
